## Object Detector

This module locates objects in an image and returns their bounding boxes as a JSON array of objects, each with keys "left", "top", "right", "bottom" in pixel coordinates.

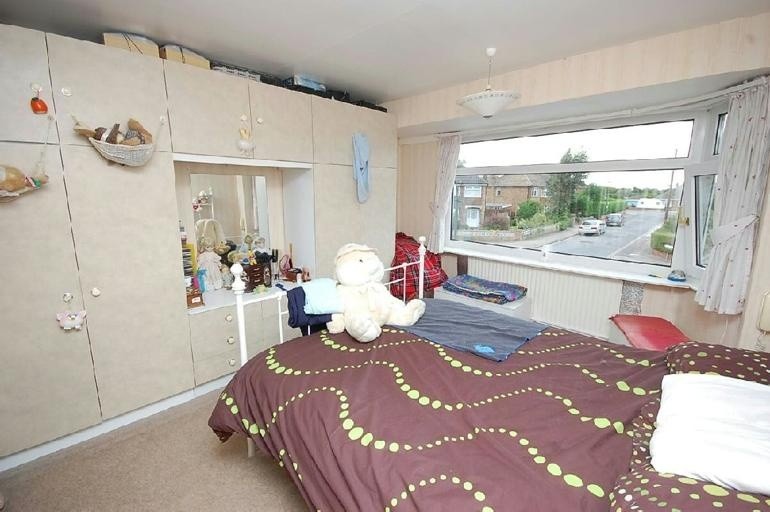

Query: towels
[
  {"left": 392, "top": 298, "right": 550, "bottom": 364},
  {"left": 286, "top": 279, "right": 345, "bottom": 338}
]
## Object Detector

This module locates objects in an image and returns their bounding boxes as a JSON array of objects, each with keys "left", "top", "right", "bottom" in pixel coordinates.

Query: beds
[{"left": 206, "top": 297, "right": 770, "bottom": 512}]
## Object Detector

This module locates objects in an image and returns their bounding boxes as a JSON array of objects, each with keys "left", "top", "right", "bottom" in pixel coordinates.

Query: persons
[{"left": 193, "top": 233, "right": 268, "bottom": 292}]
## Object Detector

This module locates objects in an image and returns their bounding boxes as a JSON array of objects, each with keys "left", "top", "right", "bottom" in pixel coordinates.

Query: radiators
[{"left": 466, "top": 257, "right": 624, "bottom": 339}]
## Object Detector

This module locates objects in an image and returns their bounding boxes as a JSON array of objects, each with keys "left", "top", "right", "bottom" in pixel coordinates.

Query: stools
[
  {"left": 432, "top": 286, "right": 532, "bottom": 321},
  {"left": 606, "top": 314, "right": 693, "bottom": 353}
]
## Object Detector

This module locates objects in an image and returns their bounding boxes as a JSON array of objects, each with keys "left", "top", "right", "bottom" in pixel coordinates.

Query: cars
[
  {"left": 606, "top": 213, "right": 624, "bottom": 227},
  {"left": 579, "top": 220, "right": 606, "bottom": 235}
]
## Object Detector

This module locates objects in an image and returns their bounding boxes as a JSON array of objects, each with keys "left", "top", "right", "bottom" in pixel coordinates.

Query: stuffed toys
[
  {"left": 56, "top": 310, "right": 87, "bottom": 330},
  {"left": 74, "top": 118, "right": 152, "bottom": 145},
  {"left": 325, "top": 243, "right": 427, "bottom": 342},
  {"left": 0, "top": 165, "right": 48, "bottom": 194}
]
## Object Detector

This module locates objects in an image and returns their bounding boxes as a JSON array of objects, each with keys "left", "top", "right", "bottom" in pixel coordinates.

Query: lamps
[{"left": 457, "top": 47, "right": 522, "bottom": 119}]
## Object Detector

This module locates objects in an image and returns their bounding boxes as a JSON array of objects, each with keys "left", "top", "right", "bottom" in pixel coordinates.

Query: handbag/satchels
[{"left": 391, "top": 233, "right": 448, "bottom": 301}]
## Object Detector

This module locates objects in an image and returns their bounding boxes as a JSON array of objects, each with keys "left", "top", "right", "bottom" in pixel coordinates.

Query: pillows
[{"left": 608, "top": 340, "right": 770, "bottom": 512}]
